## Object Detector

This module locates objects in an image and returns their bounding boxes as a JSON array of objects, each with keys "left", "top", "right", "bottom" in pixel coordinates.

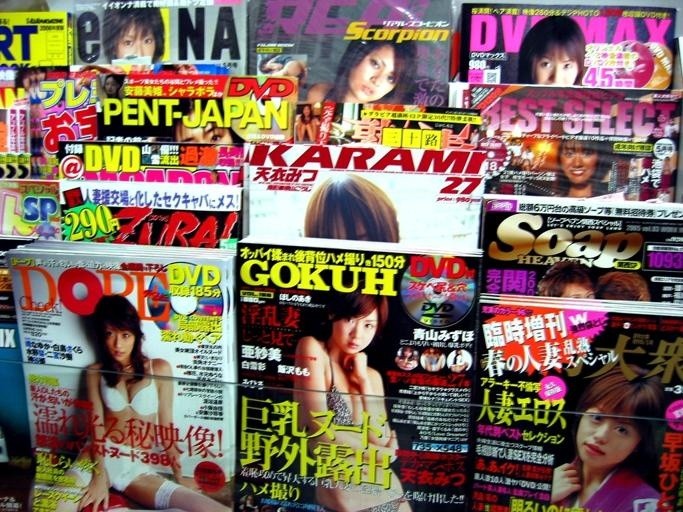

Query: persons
[
  {"left": 306, "top": 22, "right": 420, "bottom": 107},
  {"left": 510, "top": 142, "right": 532, "bottom": 171},
  {"left": 590, "top": 268, "right": 652, "bottom": 301},
  {"left": 662, "top": 156, "right": 672, "bottom": 176},
  {"left": 449, "top": 349, "right": 469, "bottom": 371},
  {"left": 103, "top": 74, "right": 120, "bottom": 98},
  {"left": 298, "top": 175, "right": 402, "bottom": 246},
  {"left": 515, "top": 15, "right": 585, "bottom": 87},
  {"left": 28, "top": 291, "right": 232, "bottom": 511},
  {"left": 295, "top": 105, "right": 320, "bottom": 145},
  {"left": 548, "top": 363, "right": 674, "bottom": 511},
  {"left": 292, "top": 283, "right": 417, "bottom": 512},
  {"left": 167, "top": 100, "right": 235, "bottom": 146},
  {"left": 422, "top": 346, "right": 444, "bottom": 373},
  {"left": 536, "top": 259, "right": 595, "bottom": 301},
  {"left": 255, "top": 55, "right": 306, "bottom": 84},
  {"left": 101, "top": 3, "right": 166, "bottom": 66},
  {"left": 14, "top": 69, "right": 51, "bottom": 102},
  {"left": 394, "top": 345, "right": 417, "bottom": 371},
  {"left": 555, "top": 131, "right": 613, "bottom": 198}
]
